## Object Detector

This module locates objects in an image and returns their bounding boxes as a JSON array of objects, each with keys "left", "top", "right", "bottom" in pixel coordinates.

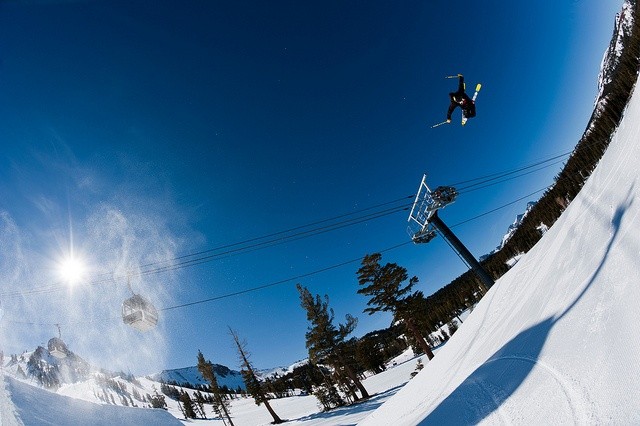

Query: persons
[{"left": 443, "top": 72, "right": 477, "bottom": 122}]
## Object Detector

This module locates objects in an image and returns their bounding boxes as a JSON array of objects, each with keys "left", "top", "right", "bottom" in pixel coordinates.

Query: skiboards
[{"left": 461, "top": 82, "right": 482, "bottom": 125}]
